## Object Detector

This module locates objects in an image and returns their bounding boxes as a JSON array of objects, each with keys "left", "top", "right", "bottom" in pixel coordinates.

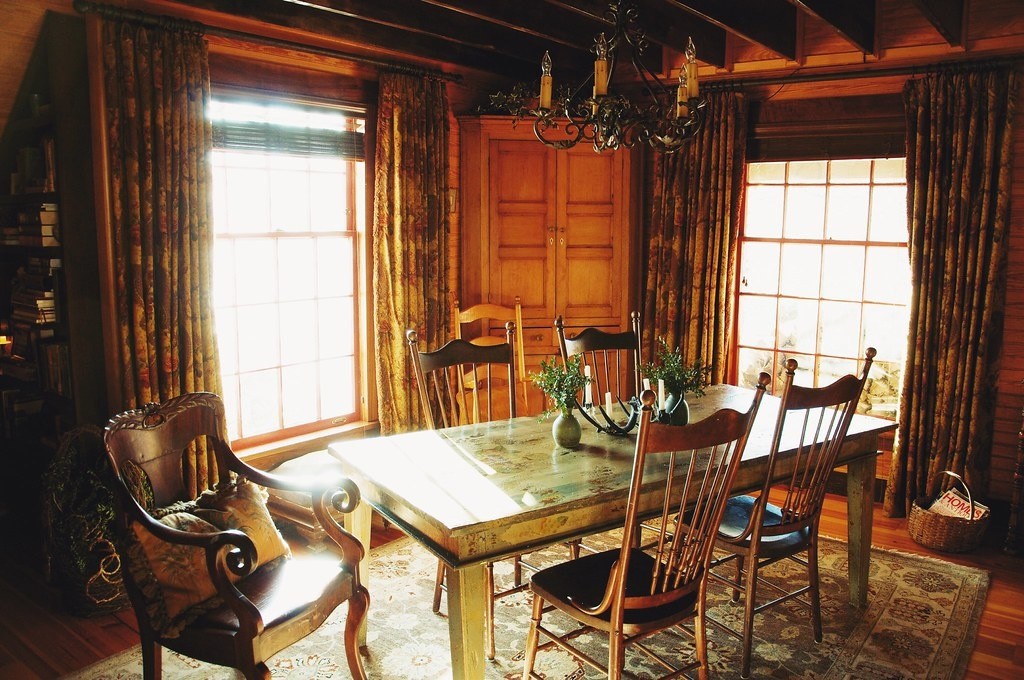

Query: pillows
[{"left": 121, "top": 475, "right": 293, "bottom": 637}]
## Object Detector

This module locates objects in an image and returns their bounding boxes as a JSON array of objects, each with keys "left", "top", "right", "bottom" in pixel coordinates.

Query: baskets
[{"left": 908, "top": 471, "right": 990, "bottom": 552}]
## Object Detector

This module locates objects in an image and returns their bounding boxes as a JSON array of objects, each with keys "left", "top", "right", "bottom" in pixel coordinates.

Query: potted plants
[
  {"left": 528, "top": 352, "right": 597, "bottom": 447},
  {"left": 635, "top": 336, "right": 713, "bottom": 426}
]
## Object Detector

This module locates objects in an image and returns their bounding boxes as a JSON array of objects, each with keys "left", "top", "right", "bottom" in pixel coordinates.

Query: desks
[{"left": 328, "top": 384, "right": 900, "bottom": 680}]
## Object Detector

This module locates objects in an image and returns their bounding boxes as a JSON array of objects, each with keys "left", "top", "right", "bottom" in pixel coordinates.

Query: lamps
[{"left": 489, "top": 1, "right": 715, "bottom": 162}]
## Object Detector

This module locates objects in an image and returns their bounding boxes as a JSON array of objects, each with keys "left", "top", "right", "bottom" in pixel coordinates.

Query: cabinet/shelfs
[
  {"left": 0, "top": 6, "right": 109, "bottom": 607},
  {"left": 459, "top": 116, "right": 632, "bottom": 422}
]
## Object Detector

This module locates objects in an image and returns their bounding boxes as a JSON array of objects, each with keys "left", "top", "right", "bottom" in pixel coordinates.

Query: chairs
[
  {"left": 673, "top": 346, "right": 877, "bottom": 679},
  {"left": 102, "top": 392, "right": 370, "bottom": 680},
  {"left": 407, "top": 321, "right": 587, "bottom": 659},
  {"left": 453, "top": 296, "right": 529, "bottom": 419},
  {"left": 555, "top": 310, "right": 684, "bottom": 556},
  {"left": 523, "top": 372, "right": 772, "bottom": 680}
]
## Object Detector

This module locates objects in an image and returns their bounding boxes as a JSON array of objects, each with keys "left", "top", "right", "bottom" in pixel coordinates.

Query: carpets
[{"left": 56, "top": 512, "right": 993, "bottom": 680}]
